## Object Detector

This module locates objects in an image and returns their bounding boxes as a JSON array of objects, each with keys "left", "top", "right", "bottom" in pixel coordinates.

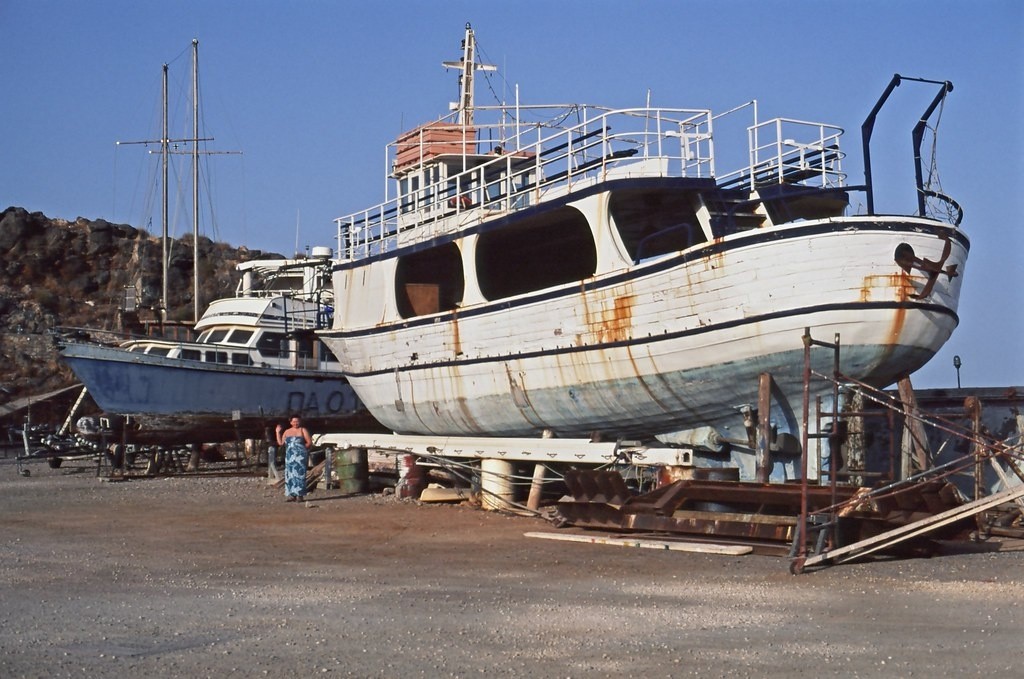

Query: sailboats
[{"left": 50, "top": 35, "right": 368, "bottom": 429}]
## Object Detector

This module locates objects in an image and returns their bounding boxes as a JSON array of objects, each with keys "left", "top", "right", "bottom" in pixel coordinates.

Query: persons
[{"left": 275, "top": 415, "right": 312, "bottom": 502}]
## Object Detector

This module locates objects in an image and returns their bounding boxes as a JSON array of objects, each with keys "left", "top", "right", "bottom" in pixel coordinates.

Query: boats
[{"left": 309, "top": 20, "right": 972, "bottom": 485}]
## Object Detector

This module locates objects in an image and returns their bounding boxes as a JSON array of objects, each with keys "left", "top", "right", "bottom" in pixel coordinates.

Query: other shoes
[
  {"left": 285, "top": 495, "right": 296, "bottom": 502},
  {"left": 296, "top": 496, "right": 304, "bottom": 502}
]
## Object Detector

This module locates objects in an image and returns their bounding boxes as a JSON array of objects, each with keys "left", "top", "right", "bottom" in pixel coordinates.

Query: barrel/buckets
[
  {"left": 335, "top": 448, "right": 370, "bottom": 494},
  {"left": 481, "top": 459, "right": 515, "bottom": 511},
  {"left": 696, "top": 468, "right": 739, "bottom": 513},
  {"left": 395, "top": 453, "right": 427, "bottom": 499}
]
